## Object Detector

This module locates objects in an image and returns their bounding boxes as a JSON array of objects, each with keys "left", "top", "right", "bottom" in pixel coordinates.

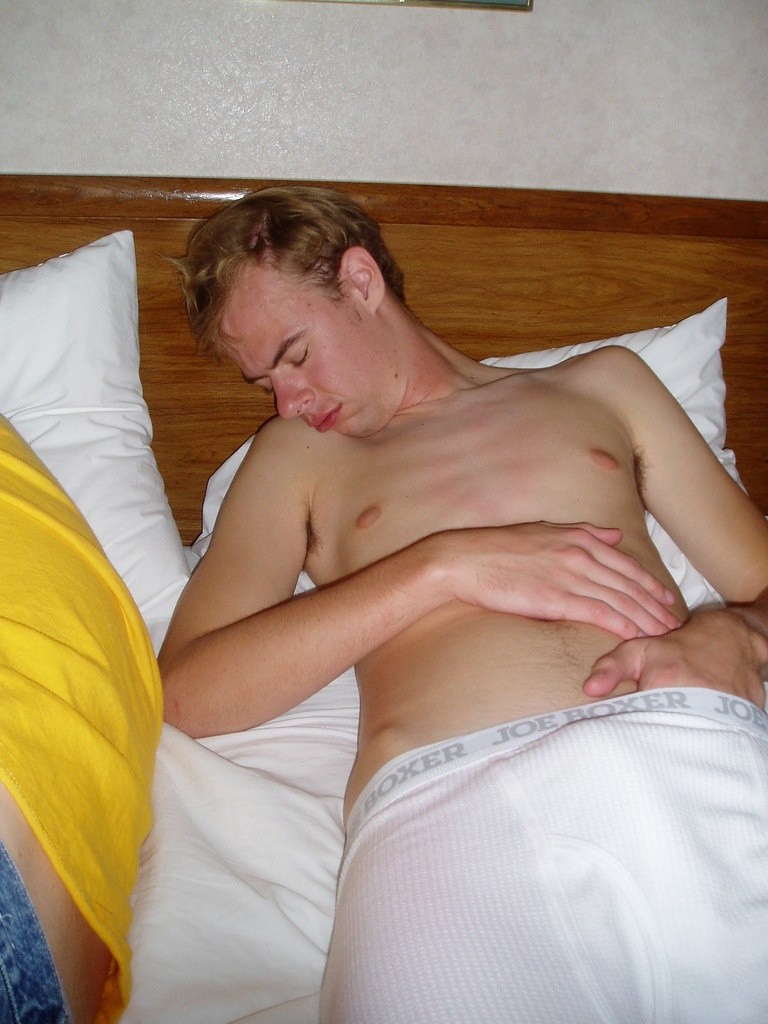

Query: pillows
[
  {"left": 197, "top": 294, "right": 751, "bottom": 611},
  {"left": 0, "top": 228, "right": 194, "bottom": 649}
]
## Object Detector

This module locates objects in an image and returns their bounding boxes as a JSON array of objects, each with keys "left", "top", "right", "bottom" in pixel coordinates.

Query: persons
[
  {"left": 157, "top": 185, "right": 768, "bottom": 1024},
  {"left": 0, "top": 410, "right": 164, "bottom": 1024}
]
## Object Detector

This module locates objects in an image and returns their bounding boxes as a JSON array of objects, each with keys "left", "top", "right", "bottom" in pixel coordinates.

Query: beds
[{"left": 0, "top": 172, "right": 768, "bottom": 1024}]
